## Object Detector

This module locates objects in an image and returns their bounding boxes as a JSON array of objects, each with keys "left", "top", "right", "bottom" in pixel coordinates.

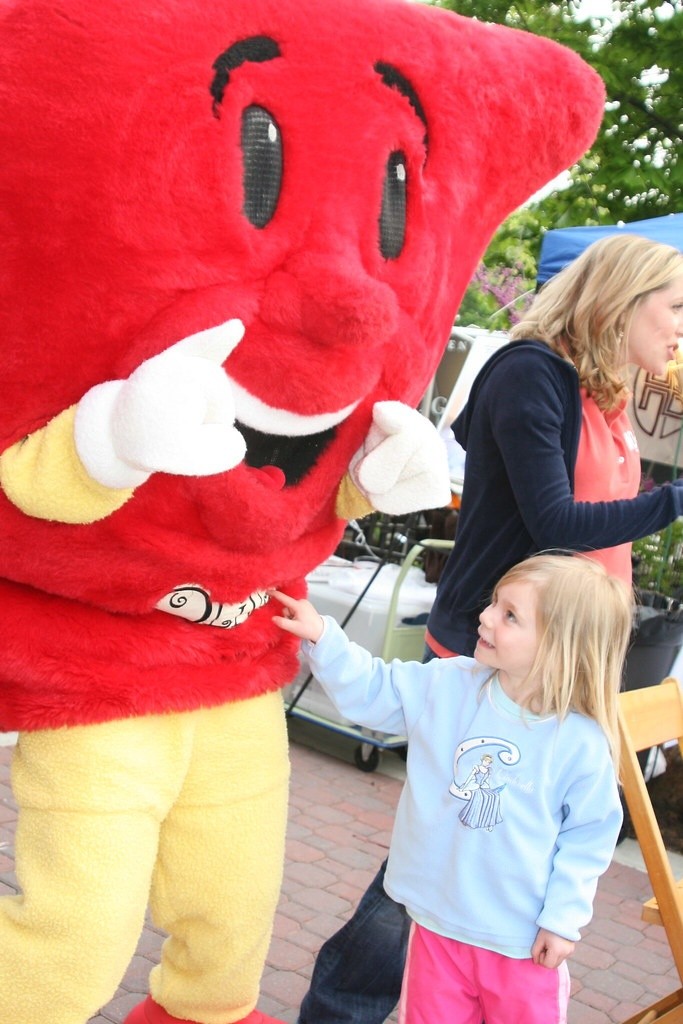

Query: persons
[
  {"left": 298, "top": 236, "right": 683, "bottom": 1024},
  {"left": 268, "top": 553, "right": 625, "bottom": 1024}
]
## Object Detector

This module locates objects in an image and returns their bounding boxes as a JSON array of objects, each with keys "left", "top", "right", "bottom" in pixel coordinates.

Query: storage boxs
[{"left": 284, "top": 562, "right": 437, "bottom": 727}]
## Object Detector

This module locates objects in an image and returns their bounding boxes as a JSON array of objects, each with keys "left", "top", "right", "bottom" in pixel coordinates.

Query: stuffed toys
[{"left": 0, "top": 0, "right": 607, "bottom": 1024}]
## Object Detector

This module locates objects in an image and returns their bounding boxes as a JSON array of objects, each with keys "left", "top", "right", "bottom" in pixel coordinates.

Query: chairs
[{"left": 607, "top": 673, "right": 683, "bottom": 1024}]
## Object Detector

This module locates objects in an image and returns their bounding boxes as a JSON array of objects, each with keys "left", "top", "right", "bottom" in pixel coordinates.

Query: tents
[{"left": 532, "top": 213, "right": 683, "bottom": 469}]
